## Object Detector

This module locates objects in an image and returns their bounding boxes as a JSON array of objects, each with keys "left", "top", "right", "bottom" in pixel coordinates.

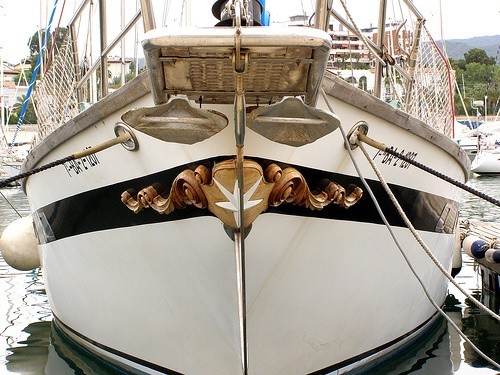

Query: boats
[
  {"left": 1, "top": 1, "right": 470, "bottom": 375},
  {"left": 469, "top": 122, "right": 500, "bottom": 175},
  {"left": 452, "top": 116, "right": 480, "bottom": 155}
]
